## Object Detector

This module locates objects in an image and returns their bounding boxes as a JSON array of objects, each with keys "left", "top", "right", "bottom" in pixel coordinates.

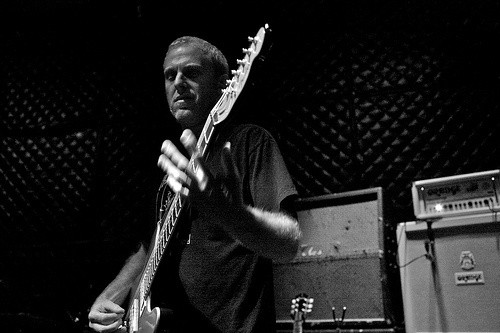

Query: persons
[{"left": 87, "top": 35, "right": 303, "bottom": 333}]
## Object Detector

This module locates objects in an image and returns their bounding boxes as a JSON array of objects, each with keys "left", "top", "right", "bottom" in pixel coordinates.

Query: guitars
[
  {"left": 290, "top": 293, "right": 314, "bottom": 333},
  {"left": 123, "top": 25, "right": 274, "bottom": 333}
]
